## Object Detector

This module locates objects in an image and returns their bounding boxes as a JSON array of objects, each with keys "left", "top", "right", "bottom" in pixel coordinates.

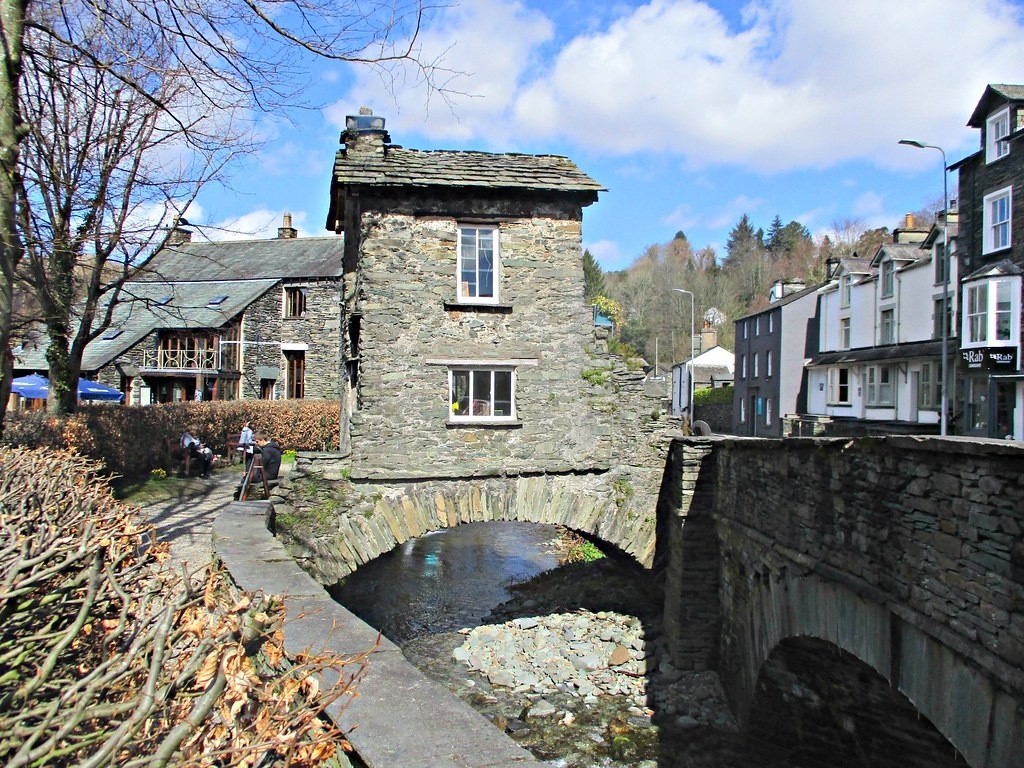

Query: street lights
[
  {"left": 897, "top": 140, "right": 953, "bottom": 438},
  {"left": 671, "top": 288, "right": 695, "bottom": 435}
]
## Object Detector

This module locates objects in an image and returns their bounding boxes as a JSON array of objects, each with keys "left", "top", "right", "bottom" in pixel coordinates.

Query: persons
[
  {"left": 179, "top": 424, "right": 213, "bottom": 480},
  {"left": 997, "top": 424, "right": 1009, "bottom": 440},
  {"left": 238, "top": 420, "right": 263, "bottom": 465},
  {"left": 234, "top": 429, "right": 281, "bottom": 502}
]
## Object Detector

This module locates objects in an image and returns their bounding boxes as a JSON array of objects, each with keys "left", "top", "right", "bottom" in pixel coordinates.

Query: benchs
[
  {"left": 228, "top": 434, "right": 250, "bottom": 465},
  {"left": 167, "top": 439, "right": 212, "bottom": 477}
]
